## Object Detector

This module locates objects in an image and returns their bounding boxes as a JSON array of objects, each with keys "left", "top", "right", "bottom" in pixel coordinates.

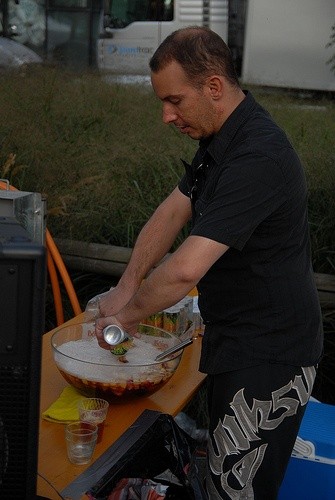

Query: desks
[{"left": 36, "top": 279, "right": 208, "bottom": 500}]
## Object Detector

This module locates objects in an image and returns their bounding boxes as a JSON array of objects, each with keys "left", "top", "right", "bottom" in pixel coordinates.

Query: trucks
[{"left": 92, "top": 0, "right": 335, "bottom": 102}]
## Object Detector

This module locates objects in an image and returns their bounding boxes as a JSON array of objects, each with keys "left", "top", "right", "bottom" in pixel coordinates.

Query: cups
[
  {"left": 74, "top": 398, "right": 109, "bottom": 444},
  {"left": 65, "top": 422, "right": 98, "bottom": 465}
]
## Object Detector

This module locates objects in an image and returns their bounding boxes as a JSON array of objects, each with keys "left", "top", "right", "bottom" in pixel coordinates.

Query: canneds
[
  {"left": 137, "top": 298, "right": 192, "bottom": 339},
  {"left": 102, "top": 324, "right": 133, "bottom": 356}
]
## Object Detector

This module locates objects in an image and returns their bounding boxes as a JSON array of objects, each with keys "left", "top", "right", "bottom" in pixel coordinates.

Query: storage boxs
[{"left": 279, "top": 394, "right": 335, "bottom": 500}]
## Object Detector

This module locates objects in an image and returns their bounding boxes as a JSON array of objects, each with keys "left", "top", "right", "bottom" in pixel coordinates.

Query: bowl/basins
[{"left": 51, "top": 322, "right": 184, "bottom": 403}]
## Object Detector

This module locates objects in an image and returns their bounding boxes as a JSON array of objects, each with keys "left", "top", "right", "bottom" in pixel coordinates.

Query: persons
[{"left": 95, "top": 26, "right": 324, "bottom": 500}]
